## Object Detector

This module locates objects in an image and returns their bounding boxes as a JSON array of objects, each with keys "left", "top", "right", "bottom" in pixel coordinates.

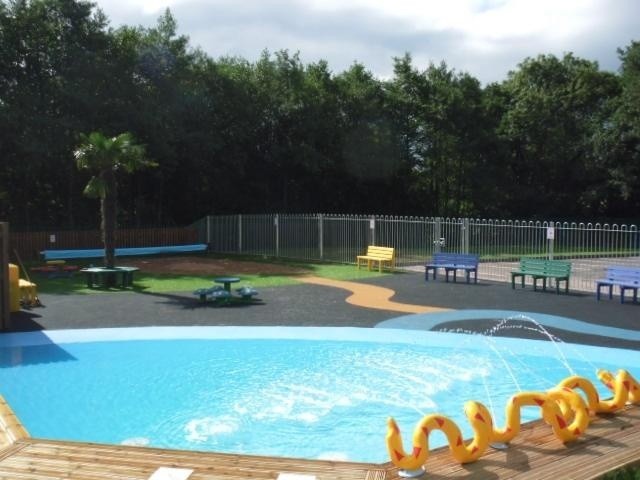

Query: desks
[{"left": 213, "top": 276, "right": 239, "bottom": 307}]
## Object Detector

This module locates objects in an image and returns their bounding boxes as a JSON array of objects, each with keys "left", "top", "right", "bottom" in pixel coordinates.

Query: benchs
[
  {"left": 198, "top": 286, "right": 258, "bottom": 305},
  {"left": 426, "top": 253, "right": 479, "bottom": 286},
  {"left": 512, "top": 258, "right": 571, "bottom": 293},
  {"left": 356, "top": 245, "right": 395, "bottom": 272},
  {"left": 596, "top": 266, "right": 639, "bottom": 305}
]
